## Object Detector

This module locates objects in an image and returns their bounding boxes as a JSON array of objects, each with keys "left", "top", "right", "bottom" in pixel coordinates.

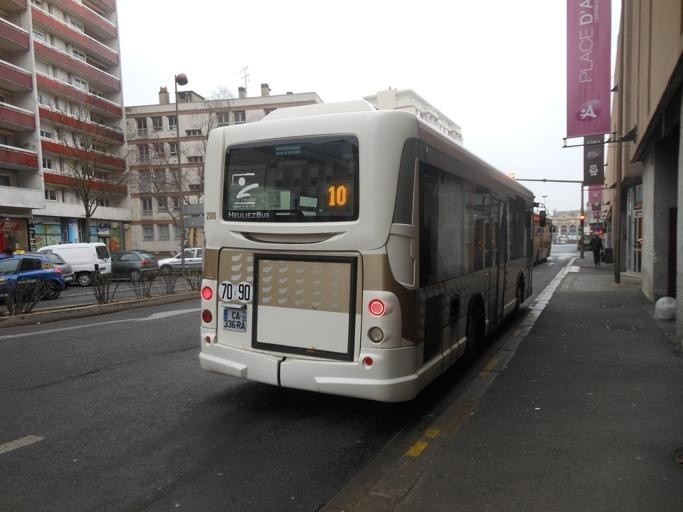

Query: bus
[
  {"left": 532, "top": 214, "right": 556, "bottom": 264},
  {"left": 197, "top": 97, "right": 546, "bottom": 405}
]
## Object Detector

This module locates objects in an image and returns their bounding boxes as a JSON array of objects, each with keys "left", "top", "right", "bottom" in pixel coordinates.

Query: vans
[{"left": 36, "top": 242, "right": 111, "bottom": 286}]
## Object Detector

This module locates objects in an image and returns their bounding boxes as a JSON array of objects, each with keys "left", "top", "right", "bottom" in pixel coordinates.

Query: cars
[
  {"left": 37, "top": 252, "right": 73, "bottom": 285},
  {"left": 0, "top": 252, "right": 65, "bottom": 300},
  {"left": 110, "top": 251, "right": 158, "bottom": 282},
  {"left": 158, "top": 248, "right": 202, "bottom": 274}
]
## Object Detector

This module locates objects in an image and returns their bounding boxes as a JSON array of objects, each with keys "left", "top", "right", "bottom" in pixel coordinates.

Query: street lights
[{"left": 172, "top": 72, "right": 188, "bottom": 276}]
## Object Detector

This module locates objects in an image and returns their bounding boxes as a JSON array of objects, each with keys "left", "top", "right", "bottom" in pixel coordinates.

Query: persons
[{"left": 591, "top": 233, "right": 603, "bottom": 268}]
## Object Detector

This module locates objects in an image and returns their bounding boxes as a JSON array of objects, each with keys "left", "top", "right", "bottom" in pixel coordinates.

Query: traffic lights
[{"left": 580, "top": 215, "right": 585, "bottom": 226}]
